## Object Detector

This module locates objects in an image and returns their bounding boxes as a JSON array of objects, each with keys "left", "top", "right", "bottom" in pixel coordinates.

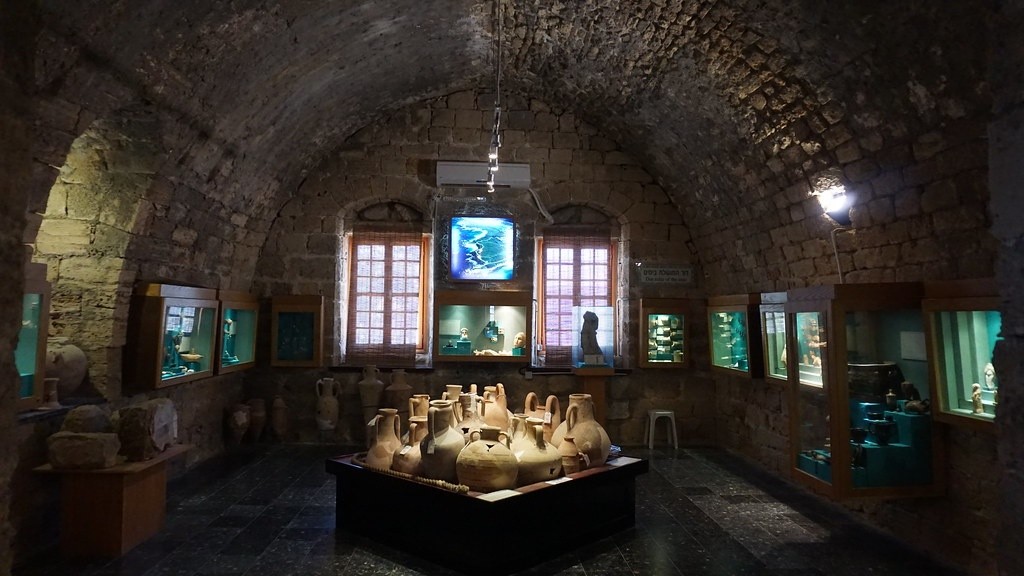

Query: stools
[{"left": 644, "top": 409, "right": 679, "bottom": 450}]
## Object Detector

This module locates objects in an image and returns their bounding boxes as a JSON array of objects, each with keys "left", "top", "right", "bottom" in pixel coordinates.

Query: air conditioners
[{"left": 436, "top": 161, "right": 532, "bottom": 188}]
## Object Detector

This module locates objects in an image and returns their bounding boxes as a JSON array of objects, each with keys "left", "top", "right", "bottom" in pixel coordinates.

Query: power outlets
[{"left": 525, "top": 371, "right": 533, "bottom": 380}]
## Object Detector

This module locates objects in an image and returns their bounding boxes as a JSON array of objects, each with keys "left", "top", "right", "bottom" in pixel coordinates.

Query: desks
[
  {"left": 324, "top": 451, "right": 649, "bottom": 576},
  {"left": 32, "top": 444, "right": 195, "bottom": 559}
]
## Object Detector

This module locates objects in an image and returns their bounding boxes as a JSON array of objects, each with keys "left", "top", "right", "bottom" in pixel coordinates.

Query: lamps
[
  {"left": 826, "top": 200, "right": 853, "bottom": 226},
  {"left": 486, "top": 0, "right": 502, "bottom": 193}
]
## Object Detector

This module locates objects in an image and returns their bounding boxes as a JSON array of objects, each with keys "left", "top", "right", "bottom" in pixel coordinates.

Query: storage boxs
[
  {"left": 443, "top": 346, "right": 457, "bottom": 354},
  {"left": 457, "top": 341, "right": 472, "bottom": 355},
  {"left": 512, "top": 348, "right": 525, "bottom": 355}
]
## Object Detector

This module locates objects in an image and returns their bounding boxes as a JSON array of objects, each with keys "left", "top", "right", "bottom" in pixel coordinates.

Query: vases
[
  {"left": 226, "top": 399, "right": 251, "bottom": 432},
  {"left": 419, "top": 400, "right": 466, "bottom": 483},
  {"left": 401, "top": 394, "right": 430, "bottom": 446},
  {"left": 384, "top": 369, "right": 413, "bottom": 413},
  {"left": 270, "top": 395, "right": 288, "bottom": 444},
  {"left": 558, "top": 436, "right": 579, "bottom": 475},
  {"left": 366, "top": 409, "right": 403, "bottom": 470},
  {"left": 500, "top": 414, "right": 529, "bottom": 450},
  {"left": 315, "top": 378, "right": 341, "bottom": 431},
  {"left": 452, "top": 394, "right": 490, "bottom": 446},
  {"left": 249, "top": 398, "right": 267, "bottom": 433},
  {"left": 391, "top": 416, "right": 429, "bottom": 476},
  {"left": 441, "top": 385, "right": 463, "bottom": 429},
  {"left": 551, "top": 394, "right": 611, "bottom": 470},
  {"left": 358, "top": 365, "right": 385, "bottom": 408},
  {"left": 524, "top": 392, "right": 561, "bottom": 443},
  {"left": 477, "top": 384, "right": 514, "bottom": 441},
  {"left": 510, "top": 418, "right": 562, "bottom": 488},
  {"left": 456, "top": 426, "right": 518, "bottom": 494}
]
